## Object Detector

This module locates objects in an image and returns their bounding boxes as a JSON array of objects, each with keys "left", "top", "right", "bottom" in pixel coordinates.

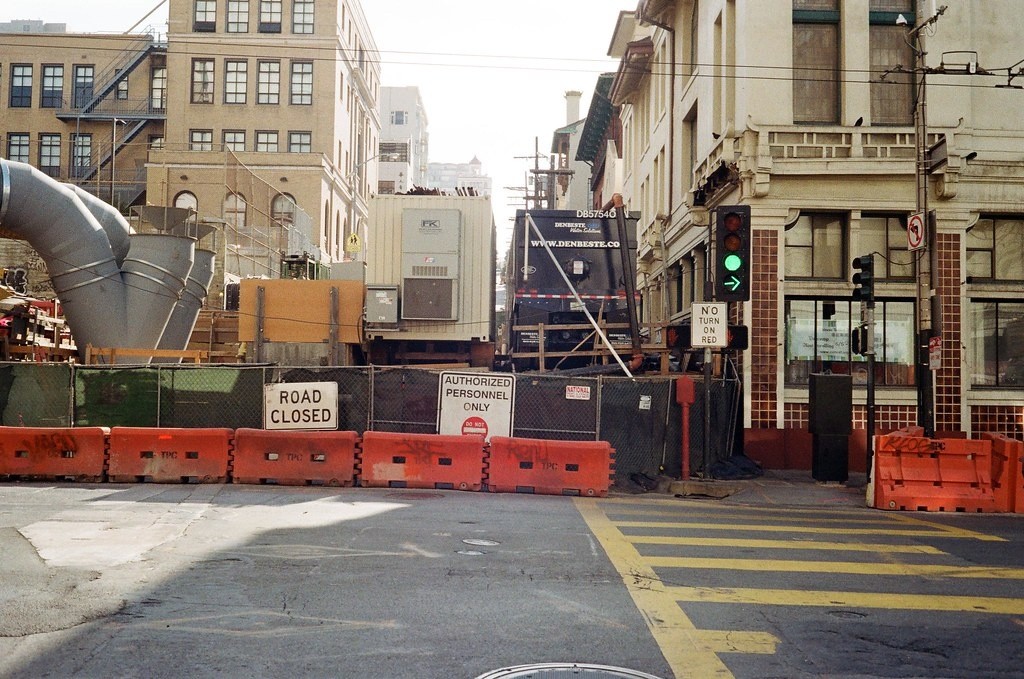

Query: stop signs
[{"left": 461, "top": 417, "right": 488, "bottom": 440}]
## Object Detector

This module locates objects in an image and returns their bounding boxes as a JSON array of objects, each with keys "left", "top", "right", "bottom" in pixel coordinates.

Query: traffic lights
[
  {"left": 852, "top": 254, "right": 874, "bottom": 302},
  {"left": 723, "top": 324, "right": 748, "bottom": 350},
  {"left": 716, "top": 205, "right": 751, "bottom": 301},
  {"left": 665, "top": 324, "right": 692, "bottom": 350}
]
